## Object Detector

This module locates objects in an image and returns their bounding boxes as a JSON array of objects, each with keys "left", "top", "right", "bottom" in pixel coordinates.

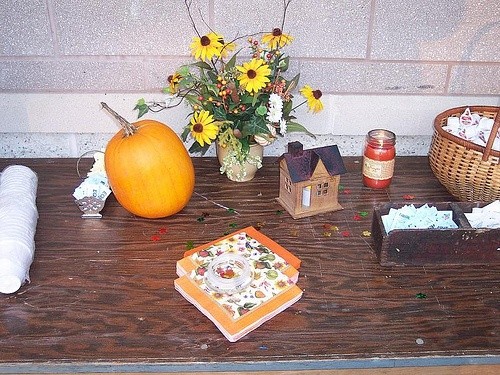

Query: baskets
[{"left": 428, "top": 104, "right": 500, "bottom": 204}]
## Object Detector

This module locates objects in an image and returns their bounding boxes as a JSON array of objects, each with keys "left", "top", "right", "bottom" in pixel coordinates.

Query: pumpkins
[{"left": 101, "top": 102, "right": 195, "bottom": 218}]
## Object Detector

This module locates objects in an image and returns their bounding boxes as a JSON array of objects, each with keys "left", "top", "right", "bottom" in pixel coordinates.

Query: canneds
[{"left": 362, "top": 129, "right": 396, "bottom": 189}]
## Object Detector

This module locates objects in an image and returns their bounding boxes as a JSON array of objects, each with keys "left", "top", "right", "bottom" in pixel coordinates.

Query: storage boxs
[{"left": 371, "top": 201, "right": 500, "bottom": 266}]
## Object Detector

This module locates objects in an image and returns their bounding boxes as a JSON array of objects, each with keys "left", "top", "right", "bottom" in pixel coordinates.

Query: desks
[{"left": 1, "top": 157, "right": 500, "bottom": 375}]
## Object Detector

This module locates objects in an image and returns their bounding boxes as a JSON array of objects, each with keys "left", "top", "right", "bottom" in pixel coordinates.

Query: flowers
[{"left": 133, "top": 29, "right": 324, "bottom": 181}]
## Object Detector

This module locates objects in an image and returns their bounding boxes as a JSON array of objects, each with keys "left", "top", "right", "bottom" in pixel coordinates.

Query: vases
[{"left": 216, "top": 141, "right": 264, "bottom": 182}]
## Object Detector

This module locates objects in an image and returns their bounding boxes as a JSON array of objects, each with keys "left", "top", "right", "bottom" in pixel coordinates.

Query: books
[{"left": 174, "top": 225, "right": 304, "bottom": 342}]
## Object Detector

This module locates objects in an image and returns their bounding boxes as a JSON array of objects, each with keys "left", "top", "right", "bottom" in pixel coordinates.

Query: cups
[
  {"left": 0, "top": 165, "right": 37, "bottom": 293},
  {"left": 363, "top": 129, "right": 395, "bottom": 188}
]
